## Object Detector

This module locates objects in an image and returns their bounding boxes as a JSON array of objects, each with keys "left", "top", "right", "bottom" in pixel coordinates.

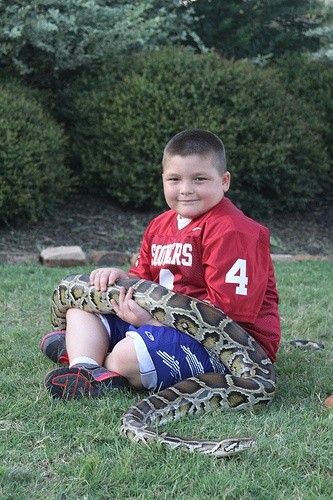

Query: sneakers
[
  {"left": 41, "top": 330, "right": 69, "bottom": 365},
  {"left": 45, "top": 363, "right": 130, "bottom": 401}
]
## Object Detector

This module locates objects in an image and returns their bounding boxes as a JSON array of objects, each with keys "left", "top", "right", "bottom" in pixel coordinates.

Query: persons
[{"left": 41, "top": 128, "right": 281, "bottom": 400}]
